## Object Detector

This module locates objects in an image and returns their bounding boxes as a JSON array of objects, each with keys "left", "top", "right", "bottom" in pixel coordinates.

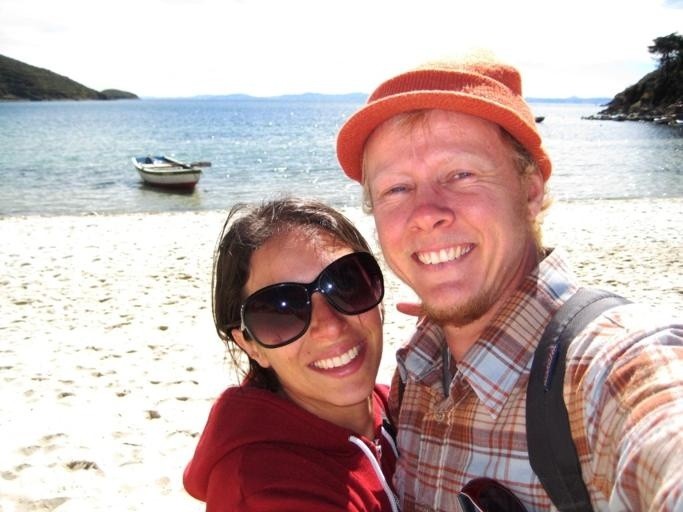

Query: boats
[{"left": 131, "top": 154, "right": 202, "bottom": 190}]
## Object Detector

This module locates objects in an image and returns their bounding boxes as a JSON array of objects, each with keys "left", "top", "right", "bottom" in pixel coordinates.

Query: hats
[{"left": 336, "top": 56, "right": 552, "bottom": 184}]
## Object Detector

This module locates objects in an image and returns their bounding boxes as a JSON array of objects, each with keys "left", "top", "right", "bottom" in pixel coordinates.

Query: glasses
[{"left": 240, "top": 251, "right": 385, "bottom": 350}]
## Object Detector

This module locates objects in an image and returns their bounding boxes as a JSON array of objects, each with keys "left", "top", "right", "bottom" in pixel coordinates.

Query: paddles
[{"left": 144, "top": 162, "right": 212, "bottom": 168}]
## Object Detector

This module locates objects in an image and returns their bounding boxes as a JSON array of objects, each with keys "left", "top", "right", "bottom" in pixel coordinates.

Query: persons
[
  {"left": 333, "top": 48, "right": 683, "bottom": 511},
  {"left": 181, "top": 196, "right": 398, "bottom": 511}
]
